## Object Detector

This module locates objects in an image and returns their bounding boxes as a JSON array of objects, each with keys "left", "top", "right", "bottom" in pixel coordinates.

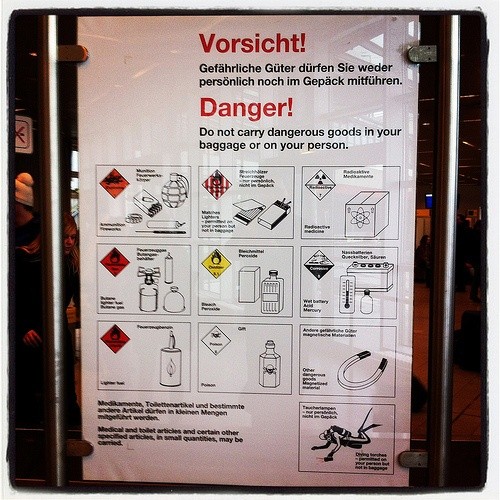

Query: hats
[{"left": 15, "top": 173, "right": 35, "bottom": 207}]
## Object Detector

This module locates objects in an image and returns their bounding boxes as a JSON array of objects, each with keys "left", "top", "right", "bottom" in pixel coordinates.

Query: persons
[
  {"left": 9, "top": 172, "right": 82, "bottom": 479},
  {"left": 413, "top": 209, "right": 486, "bottom": 302}
]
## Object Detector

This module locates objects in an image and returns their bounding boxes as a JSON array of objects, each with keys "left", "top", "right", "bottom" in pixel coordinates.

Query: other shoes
[
  {"left": 455, "top": 285, "right": 466, "bottom": 292},
  {"left": 470, "top": 295, "right": 482, "bottom": 304}
]
